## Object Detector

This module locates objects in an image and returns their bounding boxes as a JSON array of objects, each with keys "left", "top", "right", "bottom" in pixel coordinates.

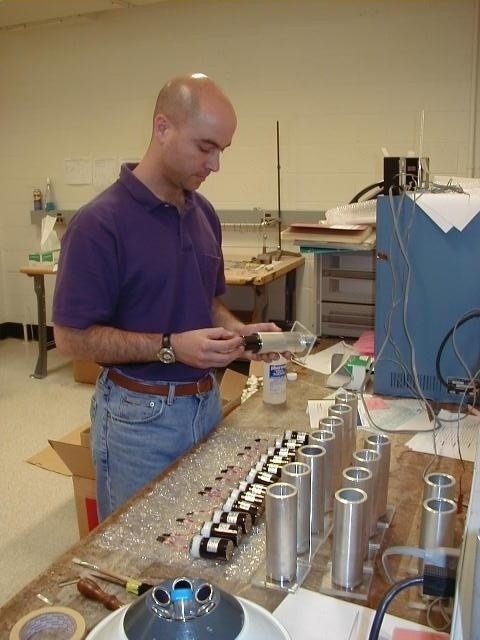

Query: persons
[{"left": 52, "top": 73, "right": 282, "bottom": 528}]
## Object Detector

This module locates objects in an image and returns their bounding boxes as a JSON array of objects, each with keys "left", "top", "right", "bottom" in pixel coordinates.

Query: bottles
[
  {"left": 44, "top": 175, "right": 56, "bottom": 214},
  {"left": 32, "top": 186, "right": 43, "bottom": 213},
  {"left": 263, "top": 352, "right": 287, "bottom": 406}
]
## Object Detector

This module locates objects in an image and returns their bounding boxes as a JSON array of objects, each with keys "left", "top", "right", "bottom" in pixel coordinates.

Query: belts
[{"left": 104, "top": 370, "right": 220, "bottom": 397}]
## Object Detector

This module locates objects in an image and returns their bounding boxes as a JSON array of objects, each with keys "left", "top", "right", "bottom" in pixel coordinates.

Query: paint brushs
[
  {"left": 88, "top": 571, "right": 148, "bottom": 596},
  {"left": 70, "top": 557, "right": 155, "bottom": 590}
]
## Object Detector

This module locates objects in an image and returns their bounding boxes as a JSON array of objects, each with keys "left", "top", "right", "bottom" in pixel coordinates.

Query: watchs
[{"left": 158, "top": 333, "right": 174, "bottom": 364}]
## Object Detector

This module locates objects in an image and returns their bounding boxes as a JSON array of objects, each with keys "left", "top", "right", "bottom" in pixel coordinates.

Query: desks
[
  {"left": 0, "top": 338, "right": 480, "bottom": 640},
  {"left": 20, "top": 239, "right": 304, "bottom": 382}
]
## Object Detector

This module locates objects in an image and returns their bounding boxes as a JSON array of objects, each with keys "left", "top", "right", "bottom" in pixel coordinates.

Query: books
[{"left": 277, "top": 223, "right": 375, "bottom": 243}]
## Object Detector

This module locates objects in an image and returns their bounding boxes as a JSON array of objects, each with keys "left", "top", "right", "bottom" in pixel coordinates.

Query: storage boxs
[{"left": 16, "top": 367, "right": 249, "bottom": 538}]
